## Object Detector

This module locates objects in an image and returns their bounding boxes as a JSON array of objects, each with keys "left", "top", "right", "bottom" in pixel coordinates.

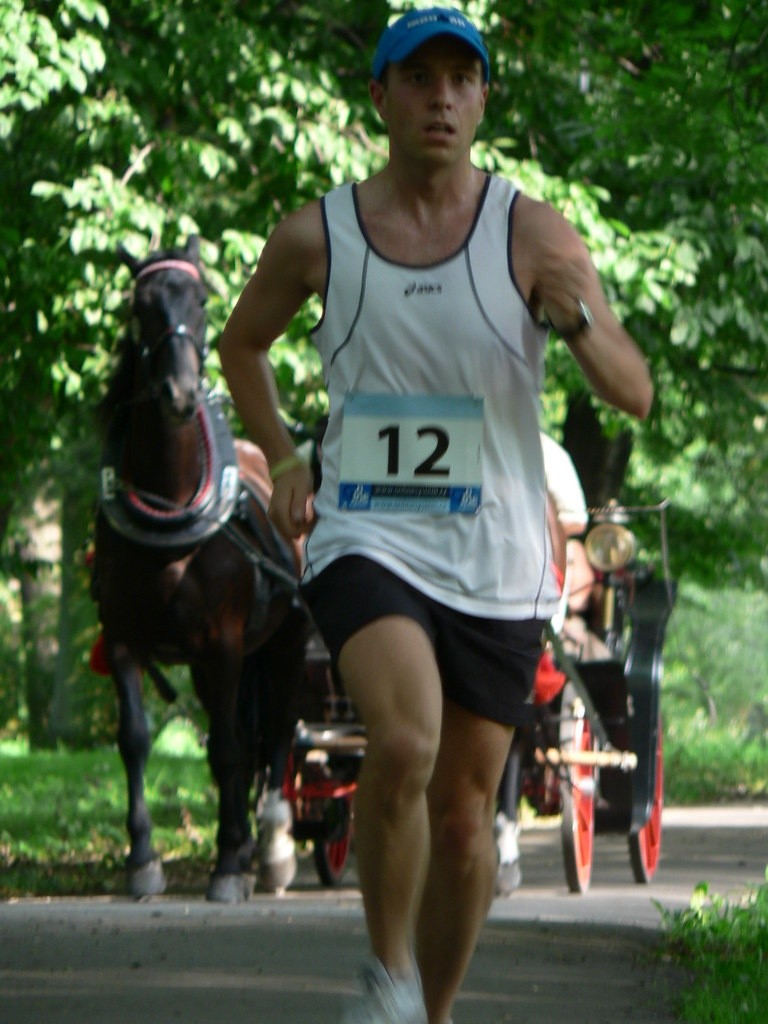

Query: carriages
[{"left": 82, "top": 227, "right": 668, "bottom": 908}]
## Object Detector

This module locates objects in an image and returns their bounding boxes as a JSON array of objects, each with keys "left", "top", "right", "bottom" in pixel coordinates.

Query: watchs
[{"left": 550, "top": 297, "right": 594, "bottom": 336}]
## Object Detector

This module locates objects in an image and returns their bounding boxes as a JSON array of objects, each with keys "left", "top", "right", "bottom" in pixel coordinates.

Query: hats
[{"left": 372, "top": 6, "right": 490, "bottom": 83}]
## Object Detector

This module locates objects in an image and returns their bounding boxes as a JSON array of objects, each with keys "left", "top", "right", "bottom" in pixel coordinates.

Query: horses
[{"left": 87, "top": 234, "right": 565, "bottom": 903}]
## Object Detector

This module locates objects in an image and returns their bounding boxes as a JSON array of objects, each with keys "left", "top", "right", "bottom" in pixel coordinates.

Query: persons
[
  {"left": 537, "top": 426, "right": 611, "bottom": 660},
  {"left": 222, "top": 7, "right": 655, "bottom": 1023}
]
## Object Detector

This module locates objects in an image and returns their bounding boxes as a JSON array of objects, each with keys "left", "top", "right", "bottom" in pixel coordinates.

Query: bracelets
[{"left": 270, "top": 452, "right": 300, "bottom": 479}]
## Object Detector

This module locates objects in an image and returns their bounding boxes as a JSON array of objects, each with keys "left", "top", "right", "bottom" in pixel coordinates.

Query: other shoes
[{"left": 341, "top": 949, "right": 428, "bottom": 1024}]
